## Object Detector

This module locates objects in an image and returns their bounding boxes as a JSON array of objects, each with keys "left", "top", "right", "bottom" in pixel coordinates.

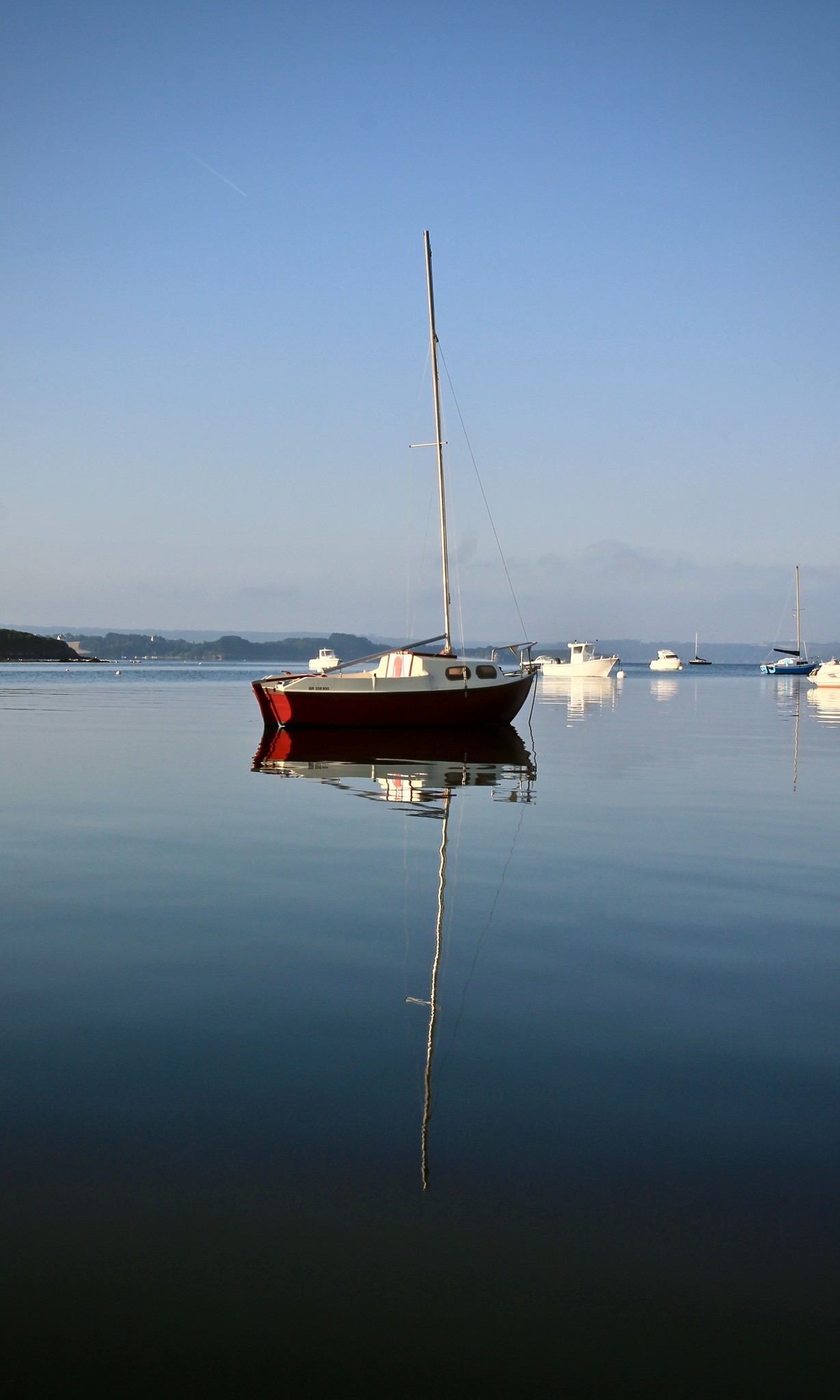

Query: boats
[
  {"left": 649, "top": 649, "right": 683, "bottom": 672},
  {"left": 308, "top": 648, "right": 342, "bottom": 670},
  {"left": 806, "top": 656, "right": 840, "bottom": 687},
  {"left": 519, "top": 639, "right": 620, "bottom": 678}
]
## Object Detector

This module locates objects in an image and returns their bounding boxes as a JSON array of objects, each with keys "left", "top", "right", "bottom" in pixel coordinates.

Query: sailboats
[
  {"left": 252, "top": 229, "right": 545, "bottom": 731},
  {"left": 759, "top": 565, "right": 820, "bottom": 675},
  {"left": 688, "top": 632, "right": 712, "bottom": 665}
]
